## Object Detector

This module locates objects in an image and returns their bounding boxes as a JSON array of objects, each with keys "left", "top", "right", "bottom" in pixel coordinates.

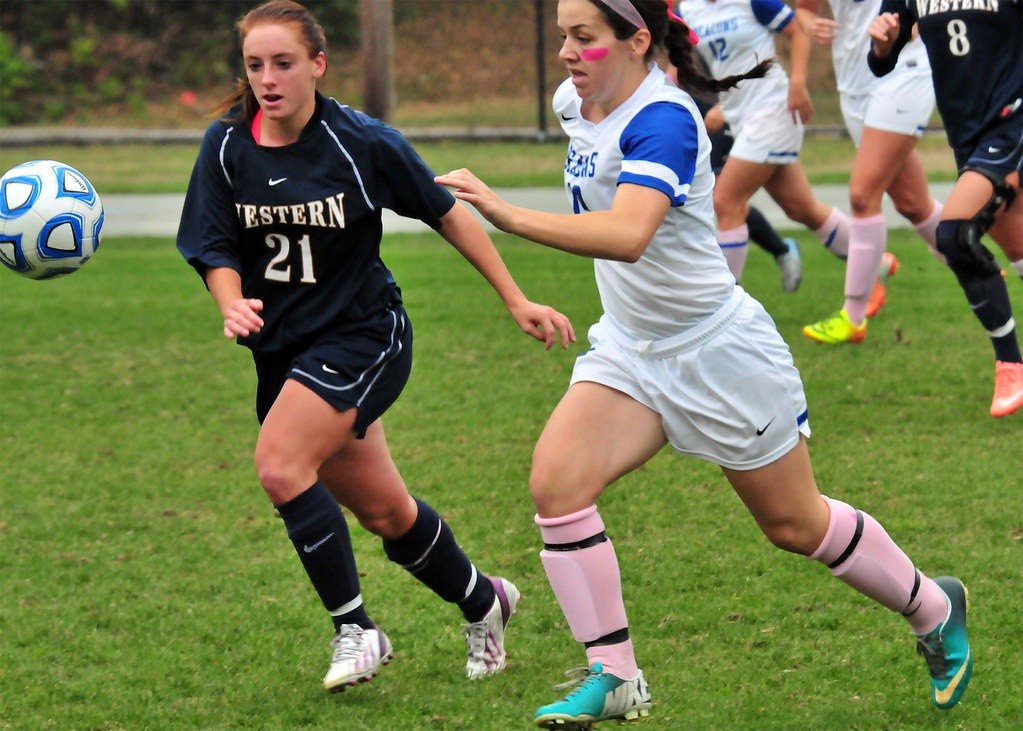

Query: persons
[
  {"left": 177, "top": 0, "right": 578, "bottom": 694},
  {"left": 659, "top": 10, "right": 802, "bottom": 295},
  {"left": 433, "top": 0, "right": 972, "bottom": 731},
  {"left": 677, "top": 0, "right": 899, "bottom": 319},
  {"left": 867, "top": 0, "right": 1023, "bottom": 417},
  {"left": 795, "top": 0, "right": 1008, "bottom": 345}
]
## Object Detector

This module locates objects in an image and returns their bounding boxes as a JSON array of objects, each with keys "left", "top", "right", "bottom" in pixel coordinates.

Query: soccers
[{"left": 1, "top": 160, "right": 105, "bottom": 281}]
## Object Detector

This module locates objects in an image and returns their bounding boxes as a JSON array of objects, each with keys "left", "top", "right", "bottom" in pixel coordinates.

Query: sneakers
[
  {"left": 321, "top": 623, "right": 393, "bottom": 694},
  {"left": 533, "top": 661, "right": 653, "bottom": 731},
  {"left": 865, "top": 251, "right": 901, "bottom": 318},
  {"left": 913, "top": 575, "right": 974, "bottom": 709},
  {"left": 776, "top": 238, "right": 801, "bottom": 292},
  {"left": 803, "top": 307, "right": 868, "bottom": 345},
  {"left": 989, "top": 359, "right": 1023, "bottom": 418},
  {"left": 460, "top": 574, "right": 520, "bottom": 680}
]
